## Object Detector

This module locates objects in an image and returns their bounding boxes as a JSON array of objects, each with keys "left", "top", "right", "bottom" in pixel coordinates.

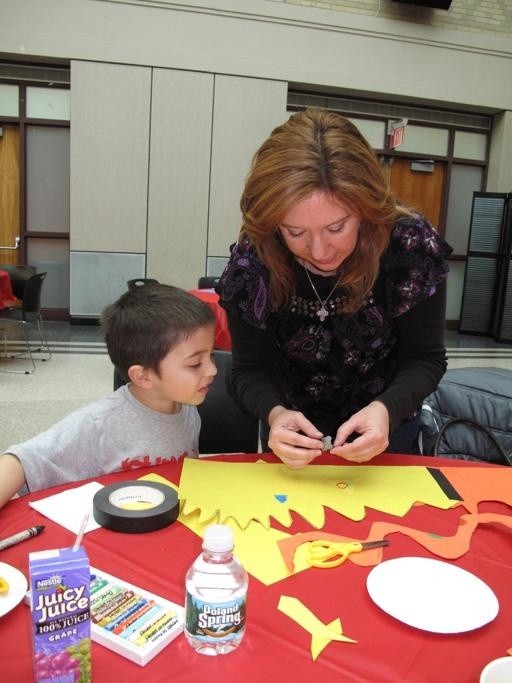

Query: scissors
[{"left": 307, "top": 541, "right": 389, "bottom": 569}]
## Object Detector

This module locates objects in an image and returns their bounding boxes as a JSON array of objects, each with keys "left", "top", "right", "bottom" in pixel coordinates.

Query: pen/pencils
[
  {"left": 0, "top": 525, "right": 44, "bottom": 549},
  {"left": 91, "top": 576, "right": 179, "bottom": 648}
]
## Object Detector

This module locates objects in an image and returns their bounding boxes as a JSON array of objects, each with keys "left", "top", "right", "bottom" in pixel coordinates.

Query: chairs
[
  {"left": 0, "top": 271, "right": 52, "bottom": 375},
  {"left": 126, "top": 277, "right": 161, "bottom": 290},
  {"left": 4, "top": 263, "right": 45, "bottom": 357},
  {"left": 113, "top": 349, "right": 258, "bottom": 455},
  {"left": 196, "top": 274, "right": 221, "bottom": 289}
]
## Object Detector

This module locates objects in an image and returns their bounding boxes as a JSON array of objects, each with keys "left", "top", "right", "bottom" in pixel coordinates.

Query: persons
[
  {"left": 0, "top": 280, "right": 220, "bottom": 513},
  {"left": 212, "top": 103, "right": 456, "bottom": 472}
]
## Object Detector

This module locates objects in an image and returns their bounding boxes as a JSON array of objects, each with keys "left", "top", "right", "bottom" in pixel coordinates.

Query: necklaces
[{"left": 294, "top": 255, "right": 346, "bottom": 323}]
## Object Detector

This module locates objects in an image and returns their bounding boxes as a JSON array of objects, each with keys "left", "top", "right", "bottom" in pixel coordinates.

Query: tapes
[{"left": 93, "top": 480, "right": 179, "bottom": 533}]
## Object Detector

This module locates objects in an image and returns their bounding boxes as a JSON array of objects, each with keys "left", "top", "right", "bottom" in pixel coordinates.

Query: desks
[
  {"left": 0, "top": 448, "right": 511, "bottom": 683},
  {"left": 188, "top": 287, "right": 233, "bottom": 351},
  {"left": 0, "top": 264, "right": 15, "bottom": 357}
]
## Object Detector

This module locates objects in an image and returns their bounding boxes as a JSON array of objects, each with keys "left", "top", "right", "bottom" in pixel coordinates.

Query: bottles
[{"left": 180, "top": 522, "right": 248, "bottom": 658}]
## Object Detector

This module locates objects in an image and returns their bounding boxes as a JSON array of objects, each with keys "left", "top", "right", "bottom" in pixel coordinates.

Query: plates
[
  {"left": 366, "top": 555, "right": 501, "bottom": 637},
  {"left": 481, "top": 655, "right": 511, "bottom": 683},
  {"left": 0, "top": 559, "right": 31, "bottom": 620}
]
